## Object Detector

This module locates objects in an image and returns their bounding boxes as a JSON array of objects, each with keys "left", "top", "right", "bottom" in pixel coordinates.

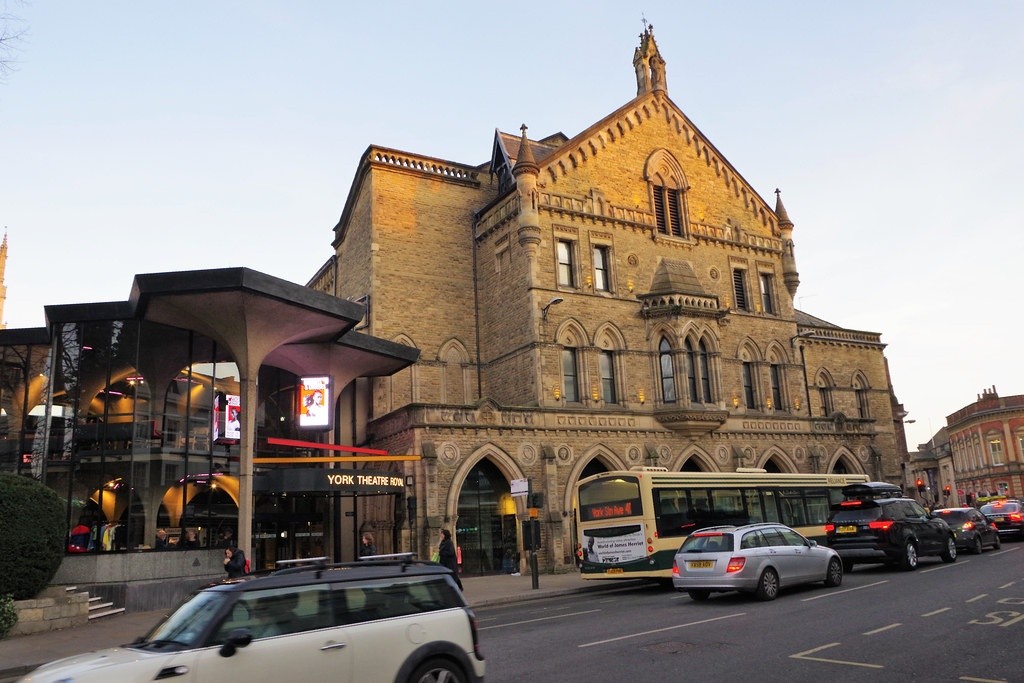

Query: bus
[
  {"left": 575, "top": 465, "right": 872, "bottom": 588},
  {"left": 970, "top": 495, "right": 1007, "bottom": 510}
]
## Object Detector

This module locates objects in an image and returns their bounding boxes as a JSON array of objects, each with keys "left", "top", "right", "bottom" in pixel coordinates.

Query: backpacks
[{"left": 243, "top": 559, "right": 250, "bottom": 574}]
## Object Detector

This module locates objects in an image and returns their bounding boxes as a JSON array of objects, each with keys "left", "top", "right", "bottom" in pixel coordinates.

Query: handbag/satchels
[{"left": 432, "top": 553, "right": 440, "bottom": 564}]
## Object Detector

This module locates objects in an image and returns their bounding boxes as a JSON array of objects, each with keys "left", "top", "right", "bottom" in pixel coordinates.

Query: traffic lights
[
  {"left": 943, "top": 486, "right": 951, "bottom": 497},
  {"left": 917, "top": 478, "right": 926, "bottom": 492},
  {"left": 1004, "top": 485, "right": 1008, "bottom": 491}
]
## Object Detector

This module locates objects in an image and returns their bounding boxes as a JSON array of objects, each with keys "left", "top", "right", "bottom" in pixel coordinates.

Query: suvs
[
  {"left": 672, "top": 522, "right": 843, "bottom": 601},
  {"left": 824, "top": 482, "right": 958, "bottom": 573},
  {"left": 15, "top": 553, "right": 486, "bottom": 683}
]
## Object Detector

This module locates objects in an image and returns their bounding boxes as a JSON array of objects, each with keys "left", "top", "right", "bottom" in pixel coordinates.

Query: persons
[
  {"left": 360, "top": 584, "right": 388, "bottom": 620},
  {"left": 438, "top": 530, "right": 463, "bottom": 592},
  {"left": 220, "top": 527, "right": 236, "bottom": 547},
  {"left": 229, "top": 408, "right": 241, "bottom": 431},
  {"left": 360, "top": 532, "right": 377, "bottom": 555},
  {"left": 223, "top": 546, "right": 246, "bottom": 579},
  {"left": 309, "top": 389, "right": 324, "bottom": 417},
  {"left": 587, "top": 535, "right": 600, "bottom": 563},
  {"left": 247, "top": 597, "right": 283, "bottom": 639},
  {"left": 303, "top": 394, "right": 314, "bottom": 417},
  {"left": 156, "top": 530, "right": 169, "bottom": 550},
  {"left": 184, "top": 527, "right": 201, "bottom": 548},
  {"left": 380, "top": 584, "right": 420, "bottom": 618}
]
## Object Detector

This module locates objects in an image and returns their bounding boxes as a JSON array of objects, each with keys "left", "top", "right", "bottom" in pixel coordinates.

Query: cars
[
  {"left": 930, "top": 508, "right": 1001, "bottom": 555},
  {"left": 979, "top": 500, "right": 1024, "bottom": 541}
]
